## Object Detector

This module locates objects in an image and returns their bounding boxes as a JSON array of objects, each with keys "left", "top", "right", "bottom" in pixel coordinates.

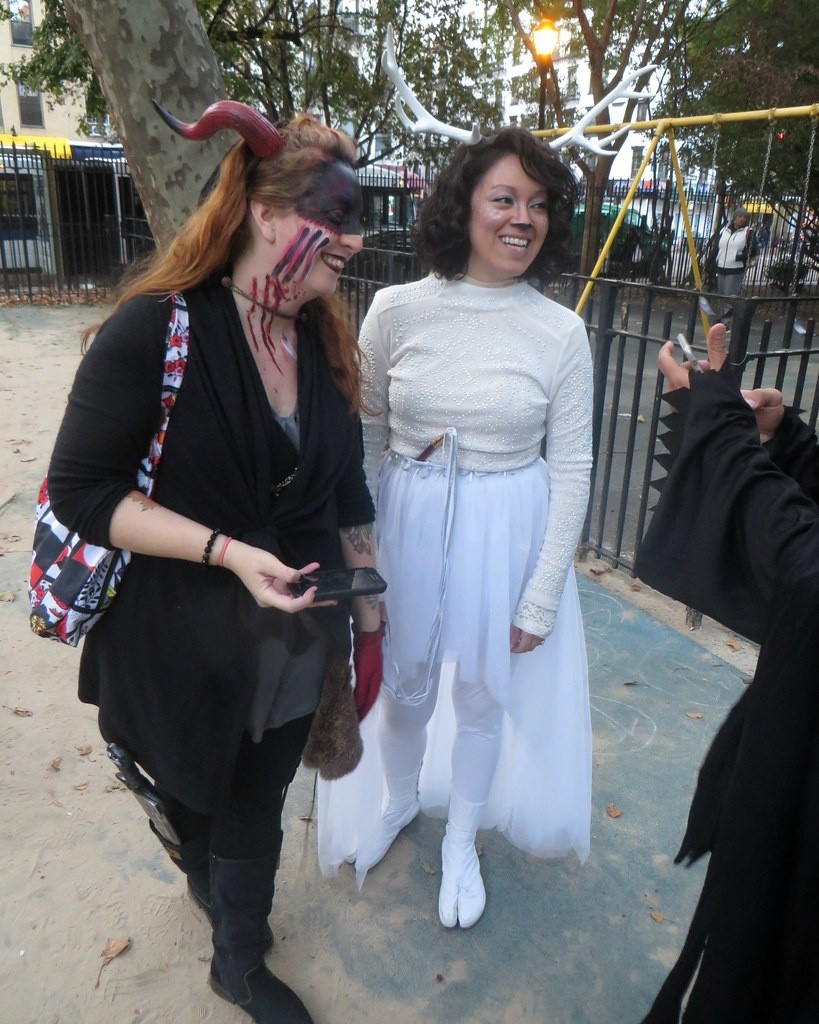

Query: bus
[{"left": 0, "top": 132, "right": 418, "bottom": 274}]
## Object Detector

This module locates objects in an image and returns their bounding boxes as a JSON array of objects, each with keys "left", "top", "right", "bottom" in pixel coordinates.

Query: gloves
[{"left": 351, "top": 620, "right": 386, "bottom": 723}]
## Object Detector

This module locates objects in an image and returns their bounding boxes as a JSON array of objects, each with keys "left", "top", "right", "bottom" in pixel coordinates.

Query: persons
[
  {"left": 706, "top": 207, "right": 759, "bottom": 333},
  {"left": 48, "top": 98, "right": 384, "bottom": 1024},
  {"left": 634, "top": 324, "right": 819, "bottom": 1024},
  {"left": 317, "top": 129, "right": 595, "bottom": 926}
]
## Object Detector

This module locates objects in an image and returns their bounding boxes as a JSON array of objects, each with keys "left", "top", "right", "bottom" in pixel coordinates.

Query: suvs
[{"left": 574, "top": 205, "right": 670, "bottom": 286}]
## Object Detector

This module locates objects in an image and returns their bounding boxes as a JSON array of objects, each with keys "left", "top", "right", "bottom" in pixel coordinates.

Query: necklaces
[{"left": 231, "top": 281, "right": 299, "bottom": 323}]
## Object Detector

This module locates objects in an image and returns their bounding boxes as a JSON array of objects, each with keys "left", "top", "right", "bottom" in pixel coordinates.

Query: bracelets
[{"left": 201, "top": 529, "right": 231, "bottom": 566}]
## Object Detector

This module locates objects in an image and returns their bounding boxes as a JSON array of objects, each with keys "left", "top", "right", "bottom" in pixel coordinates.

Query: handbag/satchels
[{"left": 26, "top": 287, "right": 189, "bottom": 648}]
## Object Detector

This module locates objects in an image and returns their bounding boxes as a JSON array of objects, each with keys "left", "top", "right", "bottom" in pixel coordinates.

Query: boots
[
  {"left": 207, "top": 850, "right": 313, "bottom": 1024},
  {"left": 149, "top": 818, "right": 274, "bottom": 952}
]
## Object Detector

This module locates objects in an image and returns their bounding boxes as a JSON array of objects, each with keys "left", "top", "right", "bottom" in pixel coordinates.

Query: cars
[{"left": 340, "top": 230, "right": 430, "bottom": 296}]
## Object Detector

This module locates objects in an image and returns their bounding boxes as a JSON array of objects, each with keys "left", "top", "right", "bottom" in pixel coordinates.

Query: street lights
[{"left": 534, "top": 18, "right": 559, "bottom": 142}]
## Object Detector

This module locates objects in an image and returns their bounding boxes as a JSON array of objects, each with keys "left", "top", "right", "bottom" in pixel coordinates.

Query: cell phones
[
  {"left": 286, "top": 568, "right": 387, "bottom": 602},
  {"left": 677, "top": 334, "right": 705, "bottom": 378}
]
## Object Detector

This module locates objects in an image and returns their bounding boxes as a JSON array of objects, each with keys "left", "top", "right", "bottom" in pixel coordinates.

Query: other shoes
[
  {"left": 343, "top": 767, "right": 421, "bottom": 870},
  {"left": 438, "top": 789, "right": 489, "bottom": 928}
]
